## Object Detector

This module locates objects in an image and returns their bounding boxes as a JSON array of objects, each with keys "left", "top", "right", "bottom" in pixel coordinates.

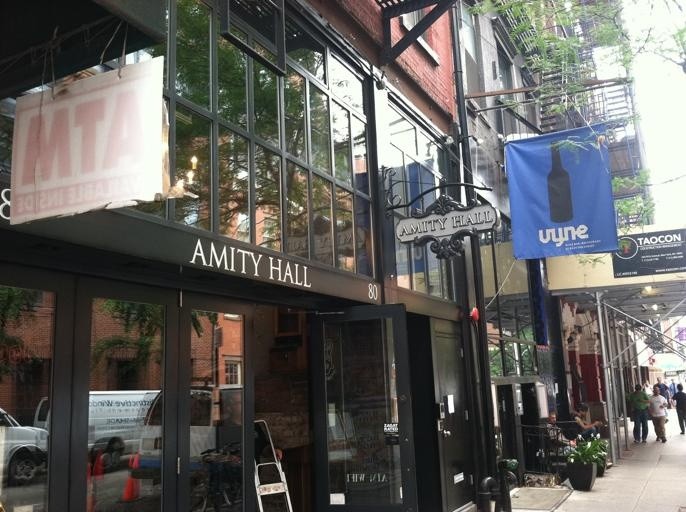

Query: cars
[{"left": 0, "top": 408, "right": 50, "bottom": 485}]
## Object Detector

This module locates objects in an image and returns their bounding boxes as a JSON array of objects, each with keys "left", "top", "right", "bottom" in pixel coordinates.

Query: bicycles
[{"left": 191, "top": 440, "right": 243, "bottom": 512}]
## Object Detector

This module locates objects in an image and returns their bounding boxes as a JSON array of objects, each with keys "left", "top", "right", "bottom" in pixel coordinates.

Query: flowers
[{"left": 565, "top": 432, "right": 608, "bottom": 469}]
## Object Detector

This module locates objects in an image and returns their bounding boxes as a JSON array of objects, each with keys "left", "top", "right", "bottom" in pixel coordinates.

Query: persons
[
  {"left": 202, "top": 391, "right": 284, "bottom": 512},
  {"left": 546, "top": 403, "right": 602, "bottom": 448},
  {"left": 629, "top": 378, "right": 686, "bottom": 442}
]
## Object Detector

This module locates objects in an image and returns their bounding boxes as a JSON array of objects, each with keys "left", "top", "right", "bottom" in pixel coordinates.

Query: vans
[{"left": 131, "top": 384, "right": 244, "bottom": 486}]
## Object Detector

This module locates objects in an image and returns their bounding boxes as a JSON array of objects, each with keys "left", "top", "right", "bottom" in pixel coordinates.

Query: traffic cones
[
  {"left": 86, "top": 448, "right": 104, "bottom": 512},
  {"left": 117, "top": 453, "right": 142, "bottom": 502}
]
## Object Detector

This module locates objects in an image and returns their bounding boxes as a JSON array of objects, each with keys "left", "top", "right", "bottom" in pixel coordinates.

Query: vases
[{"left": 566, "top": 456, "right": 605, "bottom": 491}]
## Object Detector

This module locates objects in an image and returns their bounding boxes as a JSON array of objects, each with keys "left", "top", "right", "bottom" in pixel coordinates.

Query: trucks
[{"left": 44, "top": 389, "right": 161, "bottom": 474}]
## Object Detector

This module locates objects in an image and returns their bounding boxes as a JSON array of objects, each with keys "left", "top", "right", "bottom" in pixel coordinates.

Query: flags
[{"left": 503, "top": 124, "right": 618, "bottom": 260}]
